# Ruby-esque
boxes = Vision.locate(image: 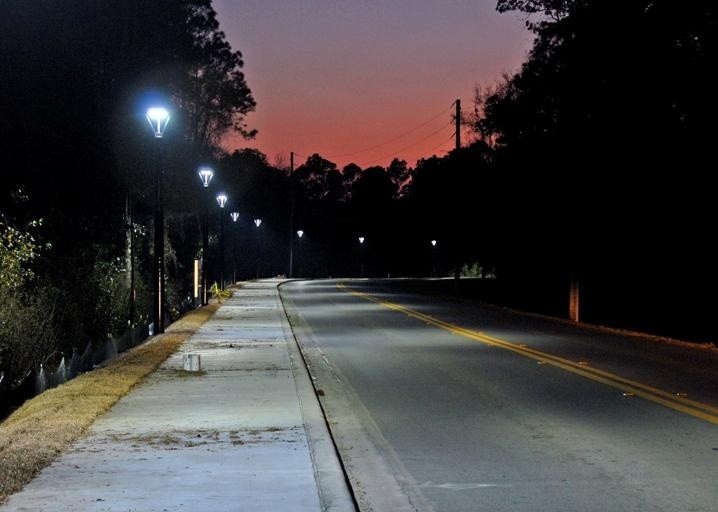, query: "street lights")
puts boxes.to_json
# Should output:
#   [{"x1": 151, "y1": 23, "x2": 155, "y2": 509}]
[
  {"x1": 430, "y1": 239, "x2": 438, "y2": 278},
  {"x1": 357, "y1": 236, "x2": 366, "y2": 278},
  {"x1": 195, "y1": 162, "x2": 213, "y2": 306},
  {"x1": 253, "y1": 215, "x2": 262, "y2": 279},
  {"x1": 297, "y1": 229, "x2": 303, "y2": 278},
  {"x1": 230, "y1": 209, "x2": 240, "y2": 285},
  {"x1": 215, "y1": 188, "x2": 227, "y2": 293},
  {"x1": 143, "y1": 105, "x2": 172, "y2": 337}
]
